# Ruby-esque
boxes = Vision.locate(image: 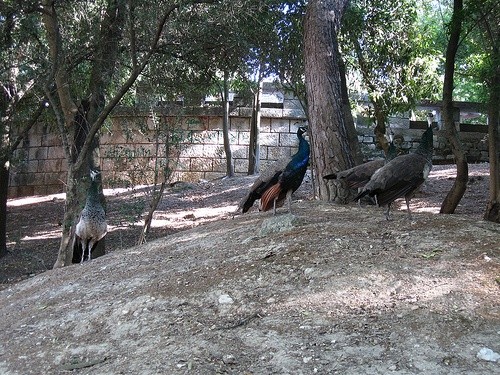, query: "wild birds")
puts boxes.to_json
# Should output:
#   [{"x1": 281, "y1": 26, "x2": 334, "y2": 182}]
[
  {"x1": 242, "y1": 121, "x2": 311, "y2": 215},
  {"x1": 72, "y1": 166, "x2": 107, "y2": 264},
  {"x1": 322, "y1": 139, "x2": 399, "y2": 208},
  {"x1": 354, "y1": 124, "x2": 433, "y2": 221}
]
